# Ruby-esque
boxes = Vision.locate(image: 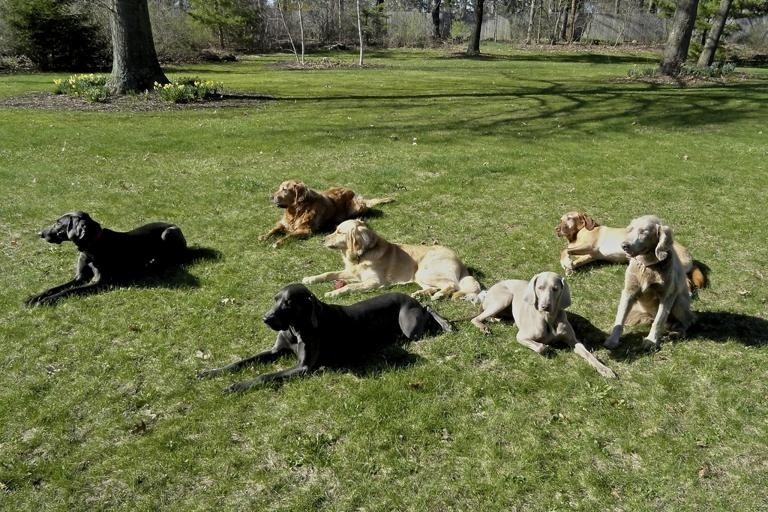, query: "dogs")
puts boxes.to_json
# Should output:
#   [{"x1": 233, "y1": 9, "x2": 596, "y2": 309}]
[
  {"x1": 195, "y1": 283, "x2": 459, "y2": 396},
  {"x1": 301, "y1": 216, "x2": 481, "y2": 303},
  {"x1": 602, "y1": 214, "x2": 697, "y2": 351},
  {"x1": 23, "y1": 211, "x2": 217, "y2": 308},
  {"x1": 462, "y1": 271, "x2": 617, "y2": 379},
  {"x1": 257, "y1": 177, "x2": 395, "y2": 249},
  {"x1": 554, "y1": 210, "x2": 704, "y2": 295}
]
